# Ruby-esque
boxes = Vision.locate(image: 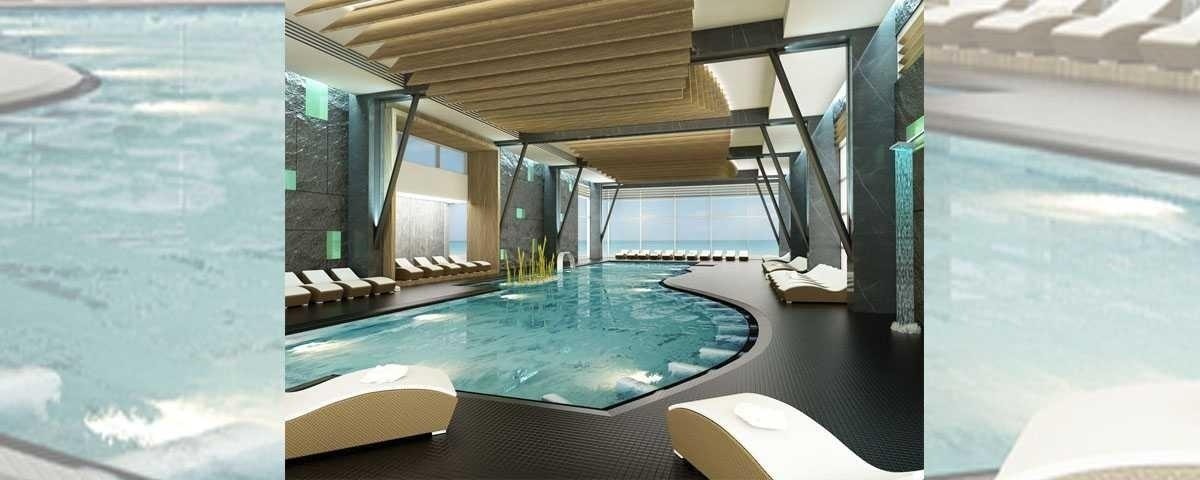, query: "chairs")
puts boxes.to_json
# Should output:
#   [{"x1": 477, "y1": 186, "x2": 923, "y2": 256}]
[
  {"x1": 762, "y1": 251, "x2": 847, "y2": 305},
  {"x1": 284, "y1": 253, "x2": 491, "y2": 309},
  {"x1": 286, "y1": 363, "x2": 460, "y2": 461},
  {"x1": 666, "y1": 392, "x2": 922, "y2": 480},
  {"x1": 615, "y1": 249, "x2": 749, "y2": 261}
]
